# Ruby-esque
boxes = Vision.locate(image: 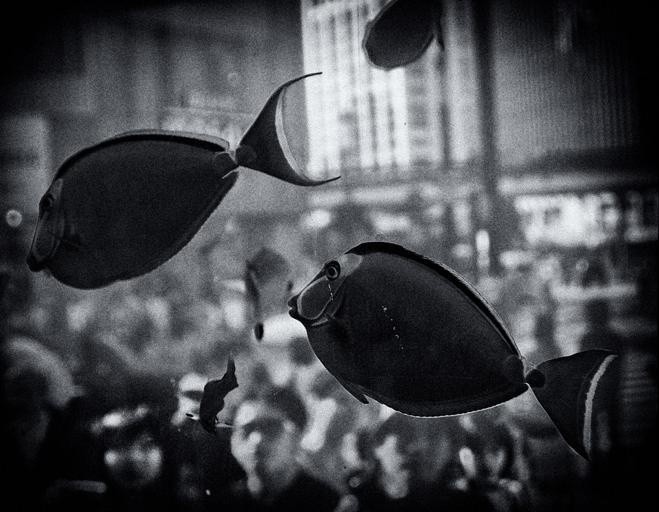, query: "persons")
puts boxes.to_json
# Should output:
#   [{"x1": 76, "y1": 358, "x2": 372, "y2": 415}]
[{"x1": 1, "y1": 241, "x2": 658, "y2": 512}]
